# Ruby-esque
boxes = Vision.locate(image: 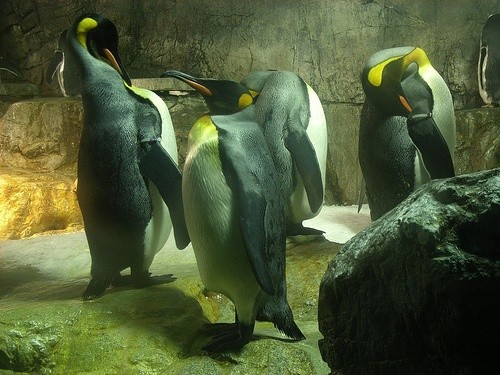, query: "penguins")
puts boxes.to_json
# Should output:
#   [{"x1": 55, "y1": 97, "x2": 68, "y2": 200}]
[
  {"x1": 50, "y1": 13, "x2": 191, "y2": 304},
  {"x1": 161, "y1": 68, "x2": 330, "y2": 356},
  {"x1": 355, "y1": 43, "x2": 458, "y2": 224}
]
